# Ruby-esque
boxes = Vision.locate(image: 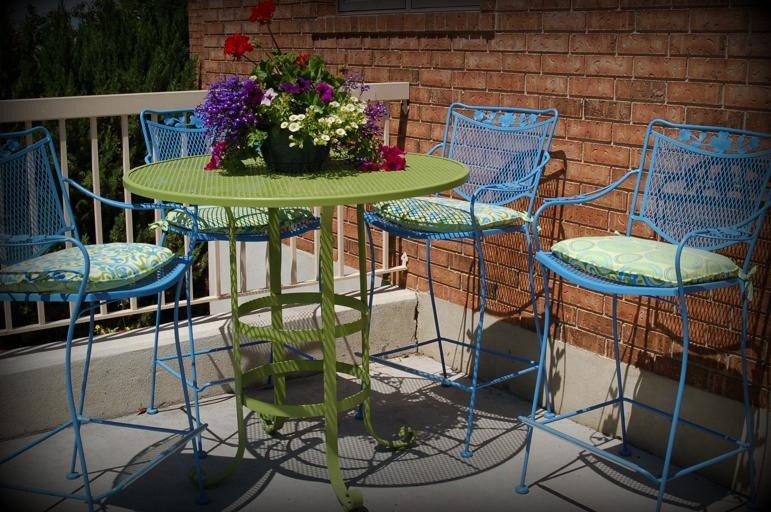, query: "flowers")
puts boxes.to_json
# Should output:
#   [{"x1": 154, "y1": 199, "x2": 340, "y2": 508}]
[{"x1": 193, "y1": 0, "x2": 408, "y2": 173}]
